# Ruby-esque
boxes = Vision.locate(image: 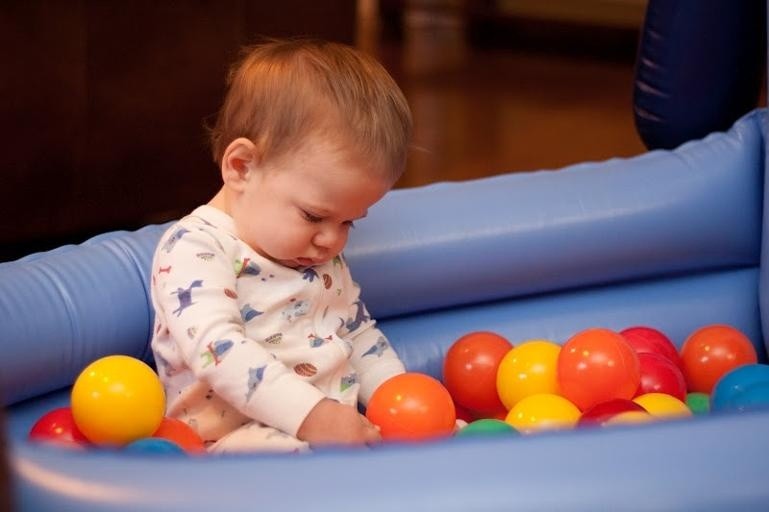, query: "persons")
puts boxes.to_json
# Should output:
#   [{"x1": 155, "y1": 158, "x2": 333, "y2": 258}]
[
  {"x1": 149, "y1": 33, "x2": 411, "y2": 459},
  {"x1": 628, "y1": 2, "x2": 768, "y2": 153}
]
